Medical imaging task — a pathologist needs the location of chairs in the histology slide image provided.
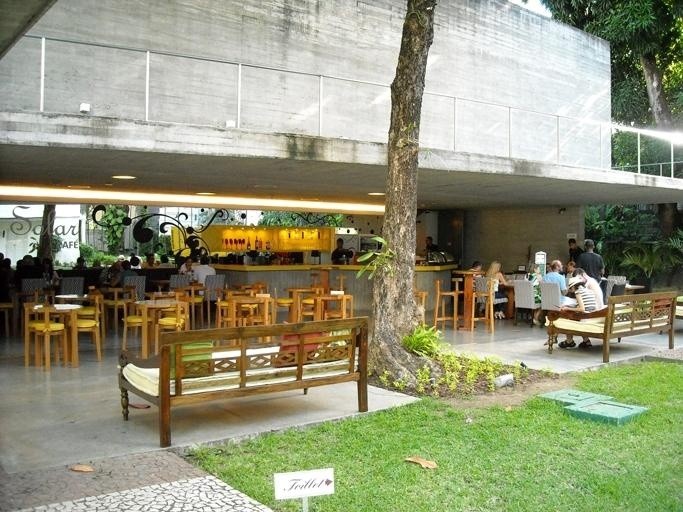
[0,274,355,373]
[471,275,627,334]
[434,280,459,332]
[414,282,429,327]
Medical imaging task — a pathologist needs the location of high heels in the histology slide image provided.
[494,311,505,319]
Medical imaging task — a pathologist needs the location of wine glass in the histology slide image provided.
[222,238,246,250]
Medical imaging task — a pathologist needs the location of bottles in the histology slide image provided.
[265,240,270,249]
[246,236,250,249]
[227,251,295,266]
[254,236,259,249]
[258,237,261,250]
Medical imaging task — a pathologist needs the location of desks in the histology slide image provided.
[454,270,486,330]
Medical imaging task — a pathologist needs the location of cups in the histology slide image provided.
[156,260,160,267]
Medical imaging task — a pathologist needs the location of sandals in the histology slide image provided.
[579,339,592,347]
[558,340,576,348]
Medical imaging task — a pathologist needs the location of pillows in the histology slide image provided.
[168,338,213,380]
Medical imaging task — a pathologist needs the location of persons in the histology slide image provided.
[426,236,439,261]
[0,248,216,320]
[524,238,606,350]
[485,259,512,320]
[331,238,353,264]
[467,260,485,317]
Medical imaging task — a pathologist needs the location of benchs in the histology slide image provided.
[118,317,369,447]
[547,291,679,363]
